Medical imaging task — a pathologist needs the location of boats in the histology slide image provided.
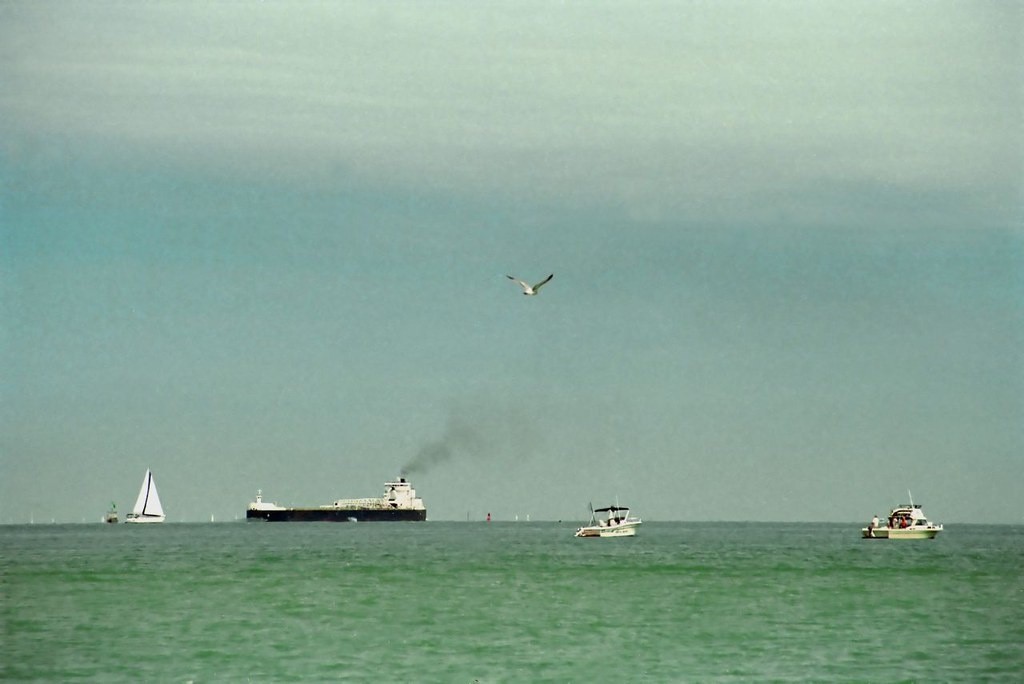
[105,502,119,524]
[573,494,643,537]
[245,476,427,522]
[861,489,944,539]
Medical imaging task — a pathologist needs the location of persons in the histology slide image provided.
[872,515,879,528]
[606,507,616,527]
[889,516,908,529]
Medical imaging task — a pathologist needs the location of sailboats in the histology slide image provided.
[125,466,166,524]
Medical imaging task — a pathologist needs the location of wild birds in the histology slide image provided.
[506,273,554,295]
